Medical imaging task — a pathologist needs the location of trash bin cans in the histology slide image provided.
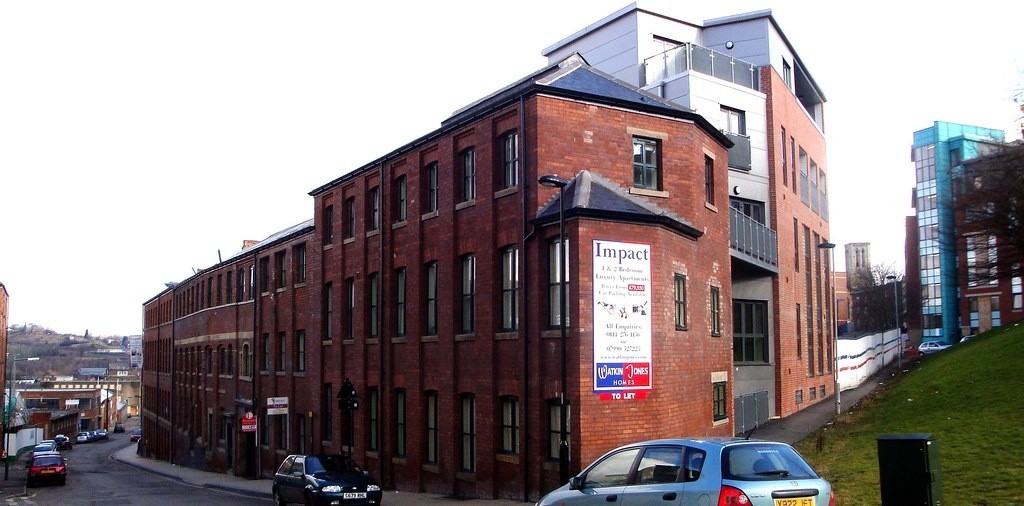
[875,432,941,506]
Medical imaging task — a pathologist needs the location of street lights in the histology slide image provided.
[886,275,902,369]
[4,357,40,481]
[817,243,841,415]
[537,175,570,487]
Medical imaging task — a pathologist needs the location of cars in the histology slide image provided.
[533,437,836,506]
[130,430,141,442]
[54,434,73,450]
[114,423,126,433]
[918,341,953,356]
[272,454,383,506]
[27,439,66,485]
[76,429,109,443]
